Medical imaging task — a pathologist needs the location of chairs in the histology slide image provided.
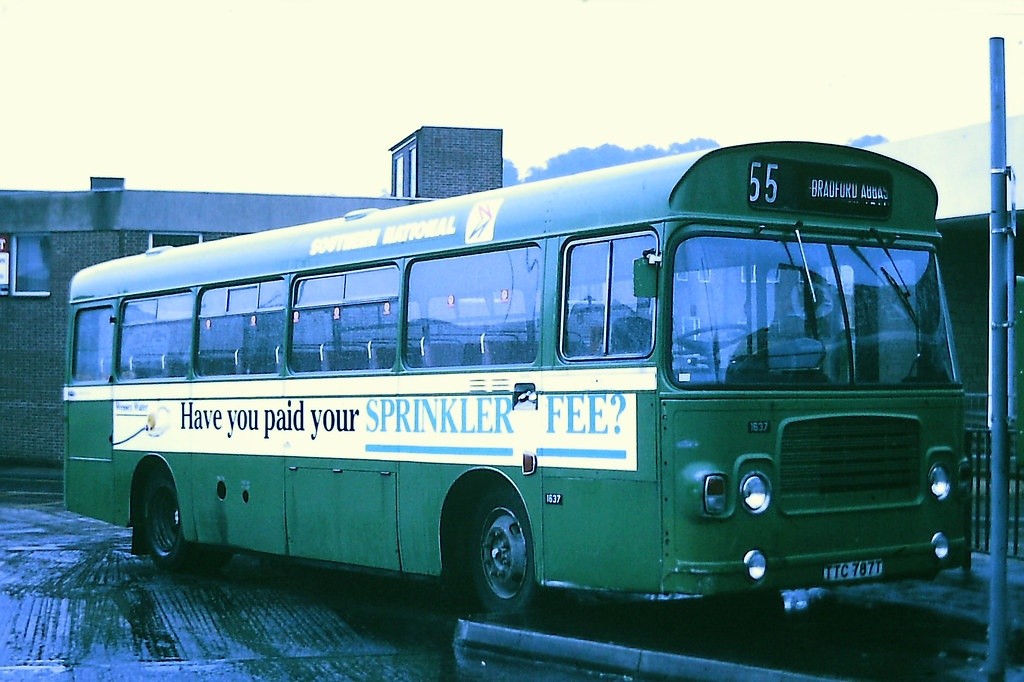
[99,292,667,381]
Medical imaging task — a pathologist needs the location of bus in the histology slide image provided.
[58,140,966,618]
